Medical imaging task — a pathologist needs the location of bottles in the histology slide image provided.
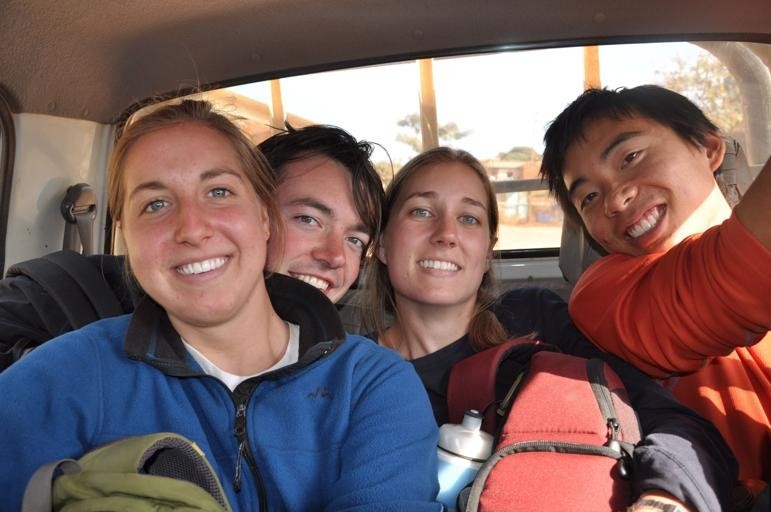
[429,410,495,511]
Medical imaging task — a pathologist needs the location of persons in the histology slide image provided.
[0,90,444,512]
[1,124,395,373]
[356,145,742,512]
[538,83,771,512]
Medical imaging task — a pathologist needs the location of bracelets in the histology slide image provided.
[637,497,682,512]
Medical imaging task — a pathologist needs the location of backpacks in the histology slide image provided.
[447,339,642,512]
[21,432,234,511]
